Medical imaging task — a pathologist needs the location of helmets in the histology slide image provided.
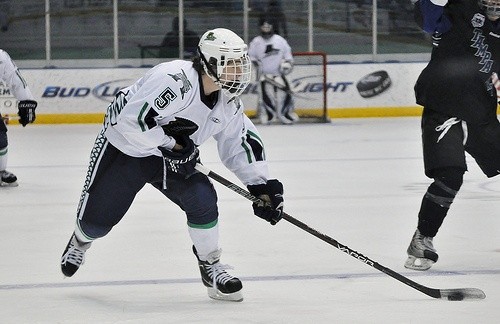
[198,28,251,97]
[258,18,275,40]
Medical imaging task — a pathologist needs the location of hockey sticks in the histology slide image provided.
[194,163,488,302]
[0,116,21,120]
[262,71,319,101]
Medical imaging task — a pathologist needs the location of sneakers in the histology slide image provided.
[0,170,18,188]
[404,230,439,269]
[192,245,244,303]
[61,232,92,276]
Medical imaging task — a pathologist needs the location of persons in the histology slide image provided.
[158,16,201,59]
[60,28,284,302]
[0,49,37,187]
[248,22,299,125]
[404,0,500,271]
[250,0,288,38]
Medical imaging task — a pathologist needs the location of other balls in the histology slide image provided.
[448,292,464,301]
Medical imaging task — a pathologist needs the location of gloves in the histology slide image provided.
[247,180,283,225]
[158,135,201,173]
[18,100,38,127]
